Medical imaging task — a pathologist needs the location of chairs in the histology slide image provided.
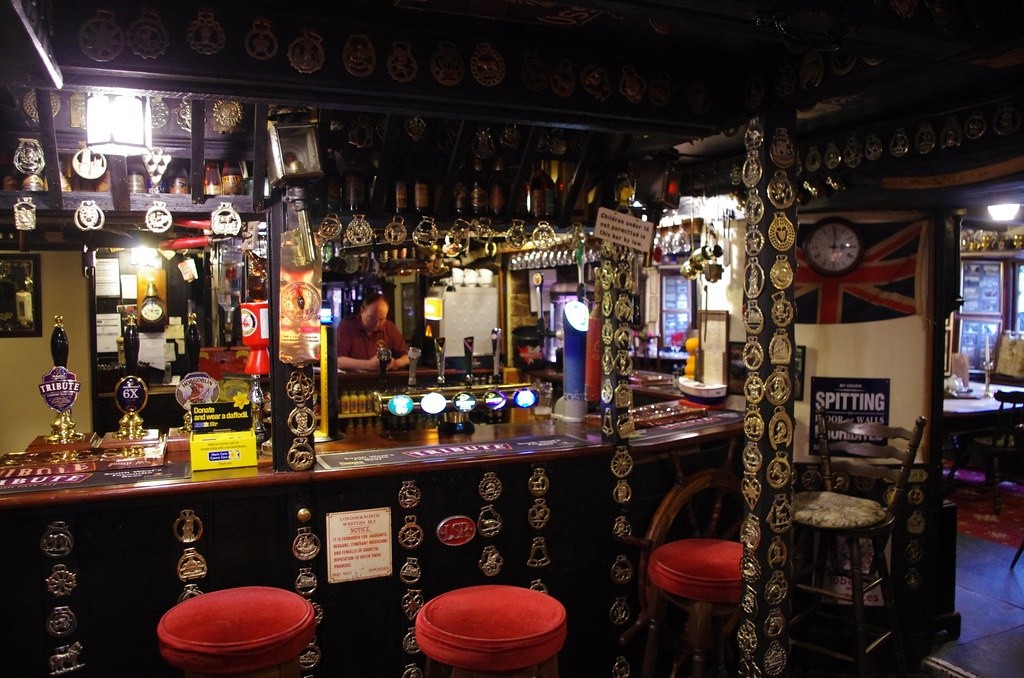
[632,334,663,372]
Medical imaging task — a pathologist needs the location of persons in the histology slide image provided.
[337,294,410,372]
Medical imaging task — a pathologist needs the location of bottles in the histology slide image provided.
[204,159,255,195]
[316,143,380,211]
[386,156,432,214]
[219,249,244,343]
[520,157,573,216]
[337,389,375,414]
[672,364,679,390]
[61,161,110,192]
[2,163,46,191]
[341,415,440,436]
[442,409,507,425]
[451,151,517,215]
[380,375,501,395]
[127,160,191,194]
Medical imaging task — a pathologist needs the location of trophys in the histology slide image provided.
[491,328,500,384]
[406,346,420,394]
[24,315,102,453]
[373,347,391,396]
[433,337,446,389]
[95,315,165,452]
[463,337,473,391]
[166,314,220,451]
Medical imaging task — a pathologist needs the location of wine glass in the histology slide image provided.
[652,218,691,256]
[508,244,578,271]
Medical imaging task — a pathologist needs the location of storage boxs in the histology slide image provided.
[189,428,258,472]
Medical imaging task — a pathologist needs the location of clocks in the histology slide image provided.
[801,216,866,278]
[140,298,166,324]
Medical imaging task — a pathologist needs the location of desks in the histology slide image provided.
[941,379,1024,452]
[631,351,687,375]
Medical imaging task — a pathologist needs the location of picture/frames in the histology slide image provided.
[957,260,1008,377]
[0,253,42,338]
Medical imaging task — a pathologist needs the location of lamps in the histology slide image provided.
[85,91,153,156]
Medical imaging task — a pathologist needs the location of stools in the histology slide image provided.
[789,413,928,678]
[416,585,567,678]
[943,391,1024,515]
[647,538,743,678]
[156,585,316,678]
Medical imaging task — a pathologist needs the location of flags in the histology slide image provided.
[793,219,924,324]
